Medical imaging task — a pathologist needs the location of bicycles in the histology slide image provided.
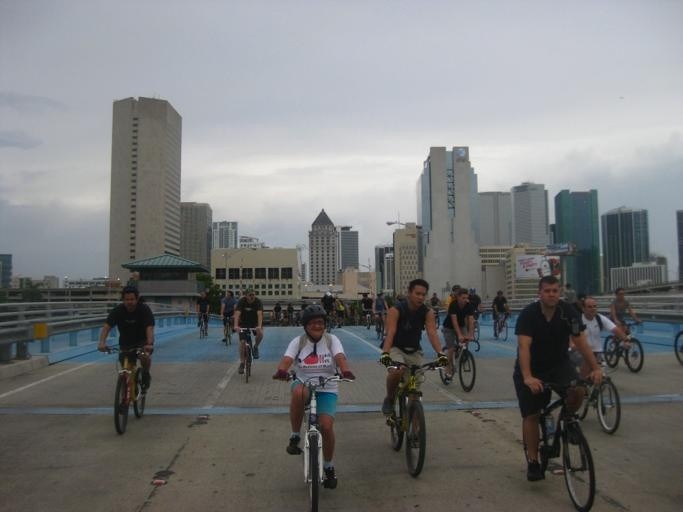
[605,320,644,373]
[378,357,440,476]
[194,310,209,340]
[494,313,510,341]
[432,309,488,394]
[269,309,388,341]
[97,344,152,434]
[231,328,256,383]
[270,373,354,512]
[220,315,234,346]
[522,345,626,511]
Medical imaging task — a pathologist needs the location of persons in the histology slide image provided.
[272,304,355,489]
[221,291,238,342]
[510,276,608,482]
[273,285,512,375]
[195,290,210,336]
[565,283,646,377]
[377,278,450,417]
[97,285,155,414]
[233,288,264,374]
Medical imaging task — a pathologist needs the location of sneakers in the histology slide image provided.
[382,398,393,413]
[253,345,259,359]
[324,466,337,489]
[287,435,302,455]
[564,423,583,444]
[239,363,245,373]
[527,459,545,481]
[141,372,150,388]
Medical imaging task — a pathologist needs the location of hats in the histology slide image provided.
[246,288,255,296]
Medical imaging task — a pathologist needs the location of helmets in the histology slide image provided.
[301,304,327,324]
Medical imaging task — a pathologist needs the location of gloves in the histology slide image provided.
[380,352,391,367]
[438,352,450,366]
[342,371,355,380]
[277,369,288,378]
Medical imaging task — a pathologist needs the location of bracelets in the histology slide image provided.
[144,342,153,345]
